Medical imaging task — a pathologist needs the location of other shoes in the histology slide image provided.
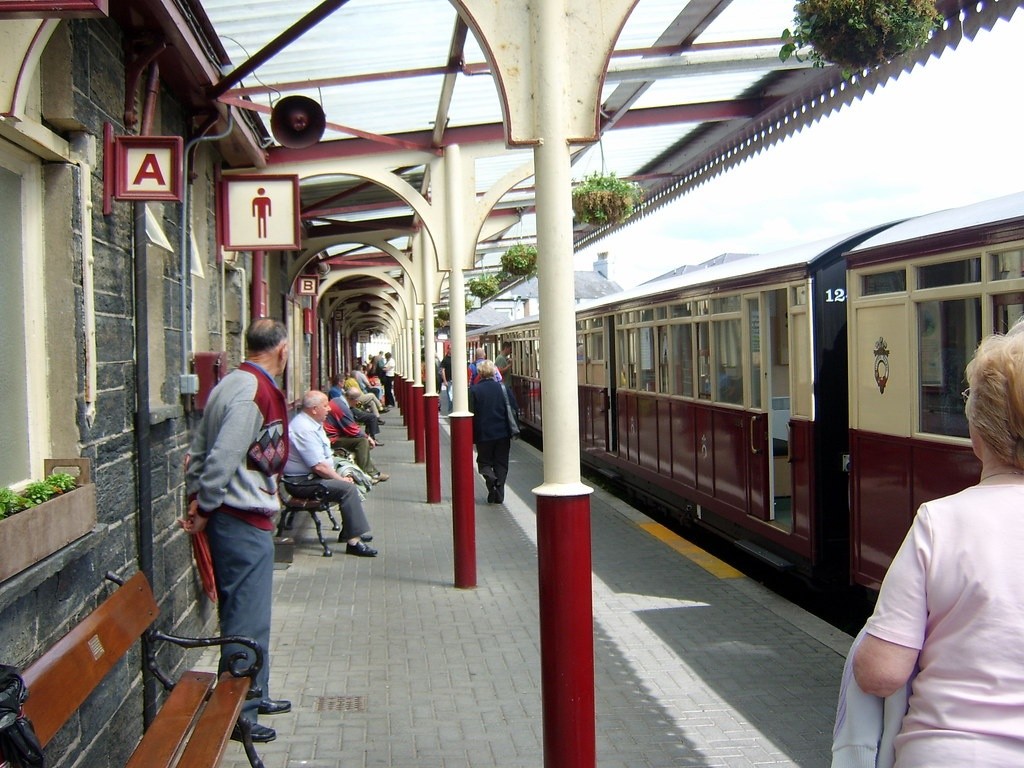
[488,485,497,503]
[375,441,384,446]
[374,473,390,481]
[370,478,379,485]
[378,419,384,425]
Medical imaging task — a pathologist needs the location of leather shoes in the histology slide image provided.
[258,698,291,714]
[360,534,373,542]
[230,723,276,742]
[346,541,378,556]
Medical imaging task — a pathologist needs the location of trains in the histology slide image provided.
[443,189,1024,596]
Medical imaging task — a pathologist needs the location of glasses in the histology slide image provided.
[962,387,972,403]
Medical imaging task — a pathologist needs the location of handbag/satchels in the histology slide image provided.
[502,383,520,436]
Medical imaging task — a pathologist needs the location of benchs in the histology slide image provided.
[0,571,263,768]
[276,448,347,556]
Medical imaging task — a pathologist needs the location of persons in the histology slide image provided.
[283,390,378,557]
[382,352,395,407]
[356,351,386,373]
[421,346,453,414]
[468,359,519,504]
[467,347,486,388]
[352,365,382,402]
[178,319,291,742]
[852,308,1024,768]
[362,366,388,410]
[344,370,391,424]
[322,387,390,486]
[495,342,526,430]
[327,374,384,446]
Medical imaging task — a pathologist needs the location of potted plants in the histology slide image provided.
[778,0,945,88]
[500,244,538,280]
[572,171,644,225]
[469,276,500,298]
[0,458,96,582]
